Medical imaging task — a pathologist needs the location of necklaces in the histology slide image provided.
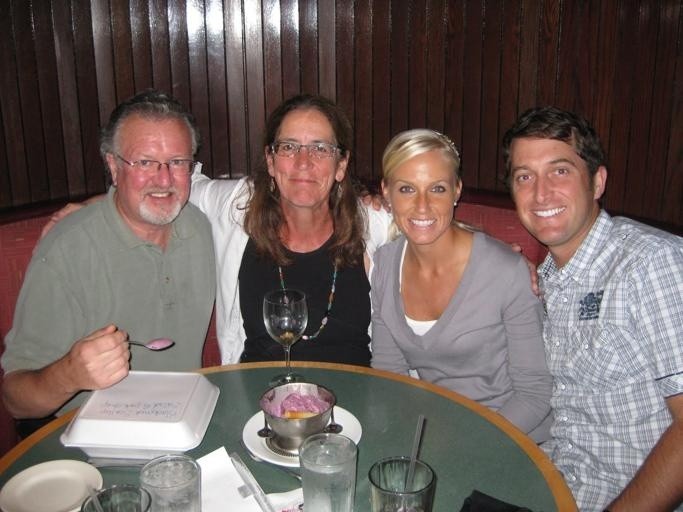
[275,262,339,341]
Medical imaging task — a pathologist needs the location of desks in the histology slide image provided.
[0,354,579,510]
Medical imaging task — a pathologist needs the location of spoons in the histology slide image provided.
[127,338,176,351]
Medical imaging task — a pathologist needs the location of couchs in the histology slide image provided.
[374,194,550,275]
[0,192,222,469]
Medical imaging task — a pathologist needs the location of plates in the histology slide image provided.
[242,406,361,468]
[0,459,102,512]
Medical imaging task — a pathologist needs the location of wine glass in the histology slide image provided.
[263,289,309,388]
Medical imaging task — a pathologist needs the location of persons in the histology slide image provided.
[0,90,391,423]
[39,91,541,433]
[368,129,552,463]
[503,105,683,512]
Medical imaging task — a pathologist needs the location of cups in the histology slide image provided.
[259,382,337,451]
[297,431,359,511]
[80,482,151,512]
[136,453,202,511]
[367,455,437,512]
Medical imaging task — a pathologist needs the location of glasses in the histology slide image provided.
[113,152,199,175]
[270,141,343,160]
